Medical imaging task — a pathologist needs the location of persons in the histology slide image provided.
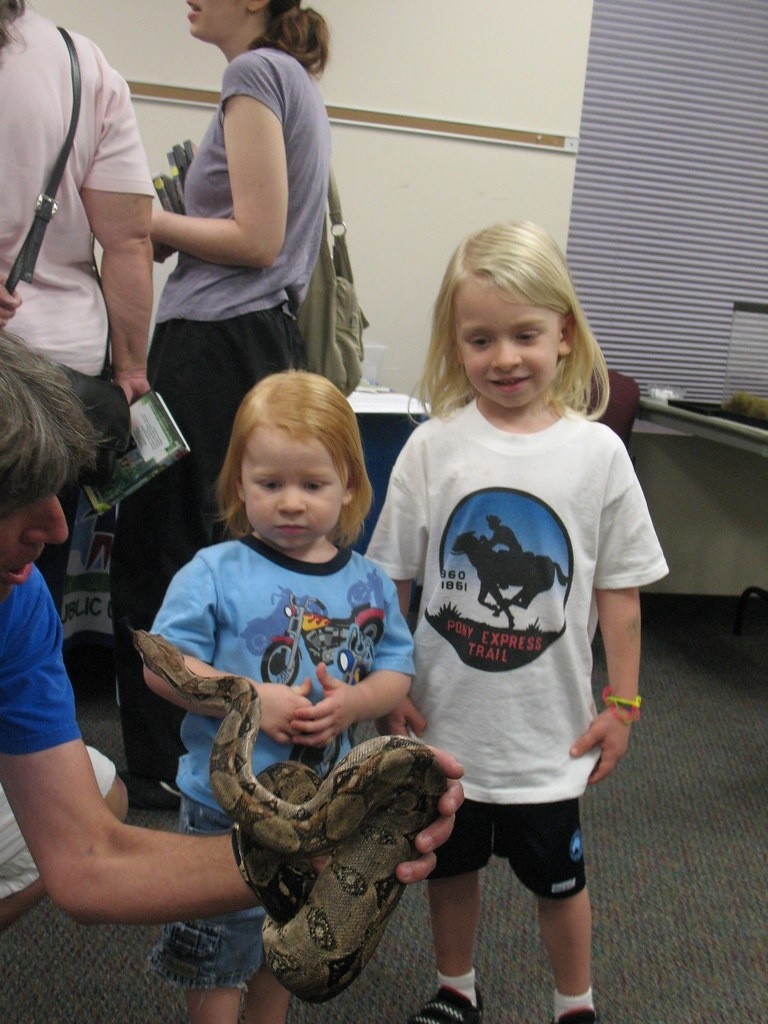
[143,363,415,1023]
[113,0,328,812]
[365,215,670,1024]
[0,0,156,602]
[0,321,466,1024]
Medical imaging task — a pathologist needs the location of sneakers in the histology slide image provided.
[408,983,482,1024]
[553,1007,595,1024]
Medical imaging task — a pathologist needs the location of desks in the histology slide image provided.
[634,397,768,636]
[40,392,433,701]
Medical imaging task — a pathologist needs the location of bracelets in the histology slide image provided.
[602,687,644,723]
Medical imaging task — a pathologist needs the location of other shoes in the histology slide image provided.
[117,771,180,810]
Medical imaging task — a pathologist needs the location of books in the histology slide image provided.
[81,391,190,517]
[154,137,197,219]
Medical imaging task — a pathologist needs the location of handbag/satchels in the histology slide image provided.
[49,362,129,489]
[296,276,370,398]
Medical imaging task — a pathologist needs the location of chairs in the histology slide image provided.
[587,368,640,447]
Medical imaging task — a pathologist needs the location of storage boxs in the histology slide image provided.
[362,332,392,385]
